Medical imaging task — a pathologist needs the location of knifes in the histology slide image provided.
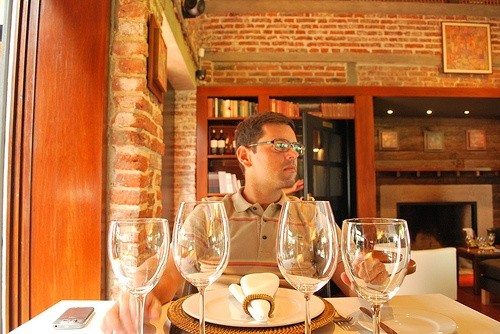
[359,307,398,334]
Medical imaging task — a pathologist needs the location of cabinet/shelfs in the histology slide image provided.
[205,96,355,196]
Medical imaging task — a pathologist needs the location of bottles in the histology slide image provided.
[217,129,225,154]
[209,129,217,154]
[232,130,237,154]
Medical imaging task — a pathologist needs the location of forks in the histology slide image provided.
[332,310,354,323]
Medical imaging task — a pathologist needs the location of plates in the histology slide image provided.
[182,285,325,329]
[357,306,458,334]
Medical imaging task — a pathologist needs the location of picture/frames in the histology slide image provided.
[153,28,168,93]
[441,22,492,74]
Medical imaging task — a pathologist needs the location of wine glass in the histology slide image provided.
[342,216,410,334]
[109,218,170,334]
[276,200,339,333]
[171,200,230,334]
[465,233,495,252]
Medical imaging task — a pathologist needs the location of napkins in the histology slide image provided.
[229,273,279,322]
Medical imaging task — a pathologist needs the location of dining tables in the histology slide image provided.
[9,247,500,334]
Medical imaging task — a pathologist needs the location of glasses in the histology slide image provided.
[245,138,305,156]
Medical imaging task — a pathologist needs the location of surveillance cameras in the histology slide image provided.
[185,0,206,16]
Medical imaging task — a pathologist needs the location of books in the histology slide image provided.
[307,102,355,118]
[207,98,300,118]
[208,170,242,194]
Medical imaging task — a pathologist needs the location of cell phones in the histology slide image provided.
[54,307,94,329]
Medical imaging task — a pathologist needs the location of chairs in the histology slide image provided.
[374,247,459,300]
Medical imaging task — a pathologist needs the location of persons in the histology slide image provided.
[281,178,304,194]
[100,112,388,334]
